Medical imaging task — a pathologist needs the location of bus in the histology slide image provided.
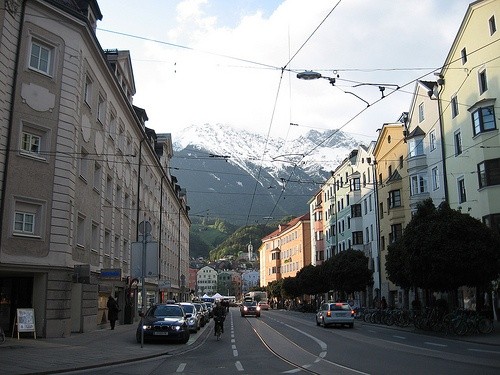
[244,292,267,303]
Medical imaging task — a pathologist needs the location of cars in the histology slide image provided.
[175,303,201,333]
[240,302,260,317]
[200,303,210,325]
[316,303,355,329]
[205,302,214,319]
[258,302,269,310]
[192,303,207,329]
[136,304,189,344]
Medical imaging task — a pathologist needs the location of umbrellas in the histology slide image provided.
[198,293,228,302]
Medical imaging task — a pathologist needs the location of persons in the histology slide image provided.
[106,295,120,330]
[212,303,226,336]
[434,294,448,313]
[173,295,177,303]
[220,299,230,313]
[412,295,422,311]
[463,296,471,312]
[270,299,274,305]
[380,296,388,310]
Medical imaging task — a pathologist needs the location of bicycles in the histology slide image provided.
[214,315,225,342]
[364,307,413,327]
[415,306,492,337]
